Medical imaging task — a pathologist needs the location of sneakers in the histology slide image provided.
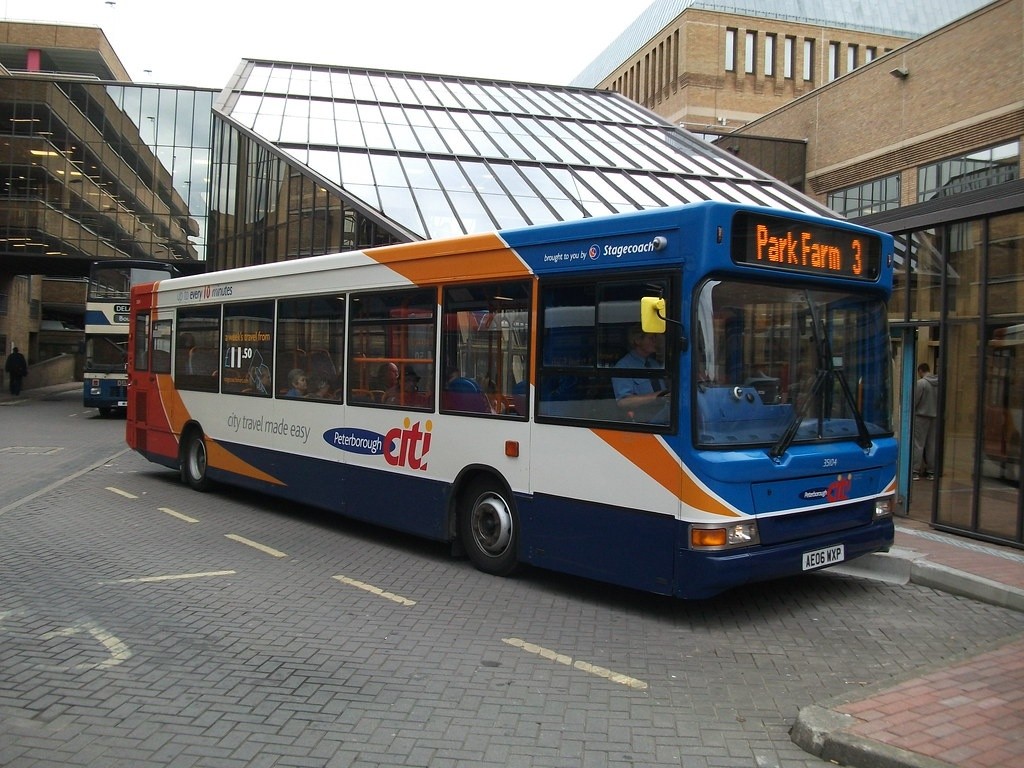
[912,473,920,481]
[925,473,935,480]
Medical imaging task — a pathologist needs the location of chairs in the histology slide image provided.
[449,377,485,413]
[306,348,337,383]
[190,345,217,375]
[277,348,306,369]
[512,380,526,416]
[141,349,169,369]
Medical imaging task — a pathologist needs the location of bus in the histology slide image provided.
[83,260,185,416]
[975,324,1024,464]
[126,202,899,600]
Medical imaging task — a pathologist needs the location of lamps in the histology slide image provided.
[889,68,909,78]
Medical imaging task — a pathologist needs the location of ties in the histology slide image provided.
[644,361,662,392]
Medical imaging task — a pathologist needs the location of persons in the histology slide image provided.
[175,334,194,374]
[349,351,369,377]
[248,366,271,394]
[373,363,399,392]
[914,363,938,481]
[286,369,308,398]
[611,323,672,422]
[396,366,422,407]
[6,347,27,395]
[308,373,330,400]
